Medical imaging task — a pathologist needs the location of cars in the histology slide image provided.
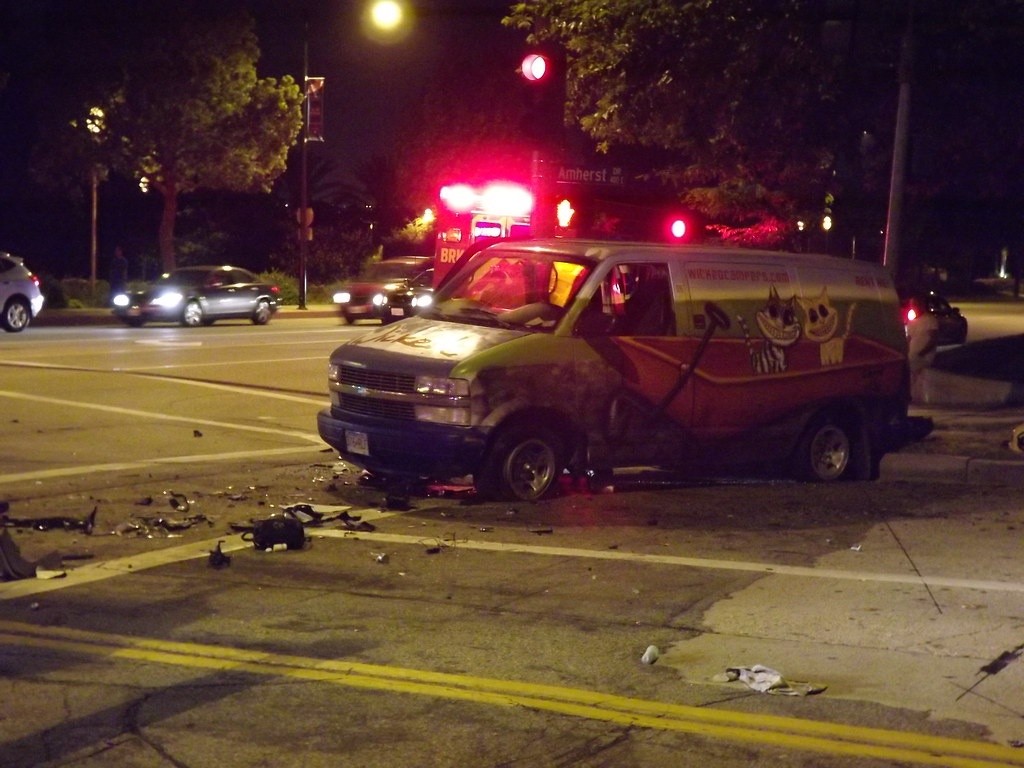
[112,263,280,328]
[328,256,437,326]
[893,291,968,357]
[1,252,45,331]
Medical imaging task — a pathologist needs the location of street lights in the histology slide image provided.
[86,104,106,295]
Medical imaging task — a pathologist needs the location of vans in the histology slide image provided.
[313,240,911,507]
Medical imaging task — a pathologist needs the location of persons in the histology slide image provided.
[106,245,129,309]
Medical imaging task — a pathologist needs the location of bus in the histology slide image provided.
[424,131,703,298]
